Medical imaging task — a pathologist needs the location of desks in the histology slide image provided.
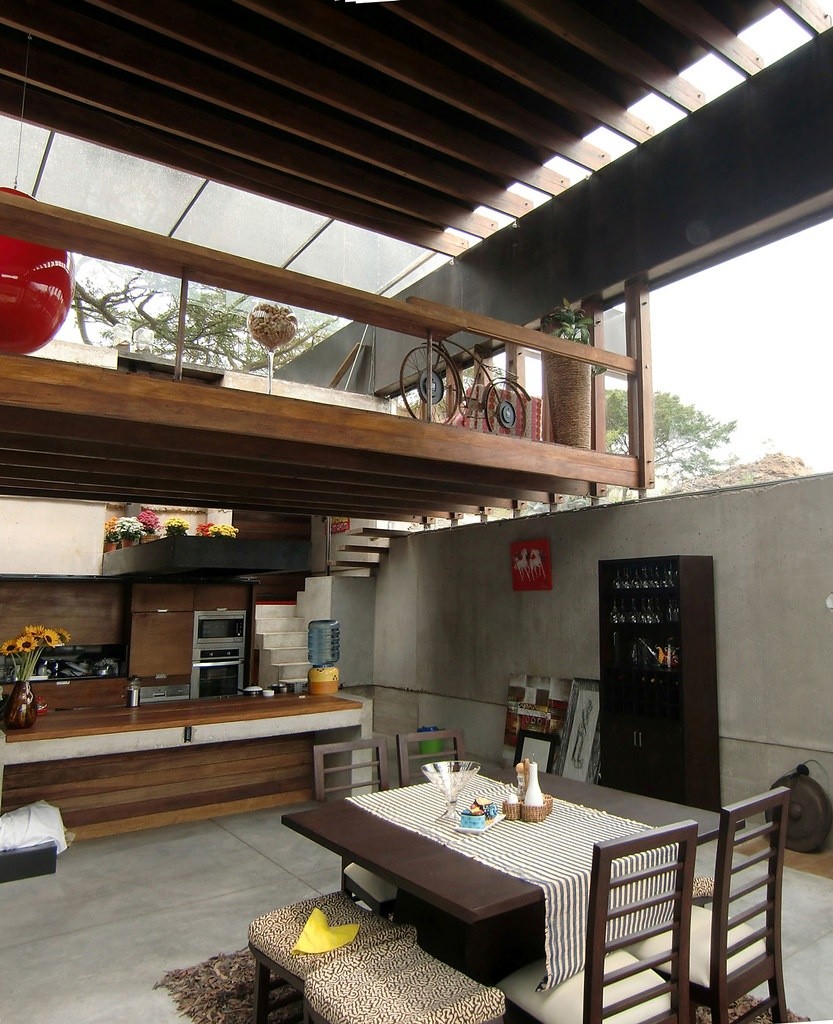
[284,768,680,989]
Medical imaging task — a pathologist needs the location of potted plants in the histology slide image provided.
[540,298,609,450]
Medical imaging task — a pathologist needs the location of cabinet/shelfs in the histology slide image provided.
[596,554,720,813]
[0,677,130,714]
[129,580,247,676]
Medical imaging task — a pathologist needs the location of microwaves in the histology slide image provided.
[192,610,246,659]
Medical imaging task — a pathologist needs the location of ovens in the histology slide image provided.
[191,660,244,699]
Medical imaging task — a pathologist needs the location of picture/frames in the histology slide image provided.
[512,676,604,786]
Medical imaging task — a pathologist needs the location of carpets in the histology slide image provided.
[154,945,814,1024]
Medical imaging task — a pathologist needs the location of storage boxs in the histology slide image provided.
[0,841,58,883]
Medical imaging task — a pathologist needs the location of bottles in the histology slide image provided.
[307,665,339,695]
[516,763,525,802]
[129,677,141,689]
[525,762,543,806]
[307,620,340,664]
[36,696,48,715]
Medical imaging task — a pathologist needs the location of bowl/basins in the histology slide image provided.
[262,690,274,696]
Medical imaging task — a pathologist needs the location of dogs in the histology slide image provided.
[515,549,545,582]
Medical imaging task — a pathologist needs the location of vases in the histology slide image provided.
[139,533,158,544]
[104,543,117,553]
[3,680,39,730]
[121,540,133,549]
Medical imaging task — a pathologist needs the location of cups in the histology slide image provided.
[127,689,140,708]
[37,666,47,676]
[418,739,442,754]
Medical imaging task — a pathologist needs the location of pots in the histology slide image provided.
[235,687,262,695]
[271,684,294,694]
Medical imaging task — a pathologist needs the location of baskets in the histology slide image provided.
[502,794,554,822]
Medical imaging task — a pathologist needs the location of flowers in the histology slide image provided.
[106,507,239,550]
[0,624,71,681]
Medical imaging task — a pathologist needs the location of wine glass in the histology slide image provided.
[421,761,481,821]
[610,564,680,625]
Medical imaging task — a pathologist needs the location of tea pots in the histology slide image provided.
[635,636,681,669]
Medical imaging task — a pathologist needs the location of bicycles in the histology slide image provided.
[398,336,530,440]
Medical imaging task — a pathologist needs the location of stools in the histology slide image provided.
[248,891,505,1024]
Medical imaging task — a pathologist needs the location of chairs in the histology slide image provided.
[312,729,466,916]
[438,384,542,442]
[500,787,790,1024]
